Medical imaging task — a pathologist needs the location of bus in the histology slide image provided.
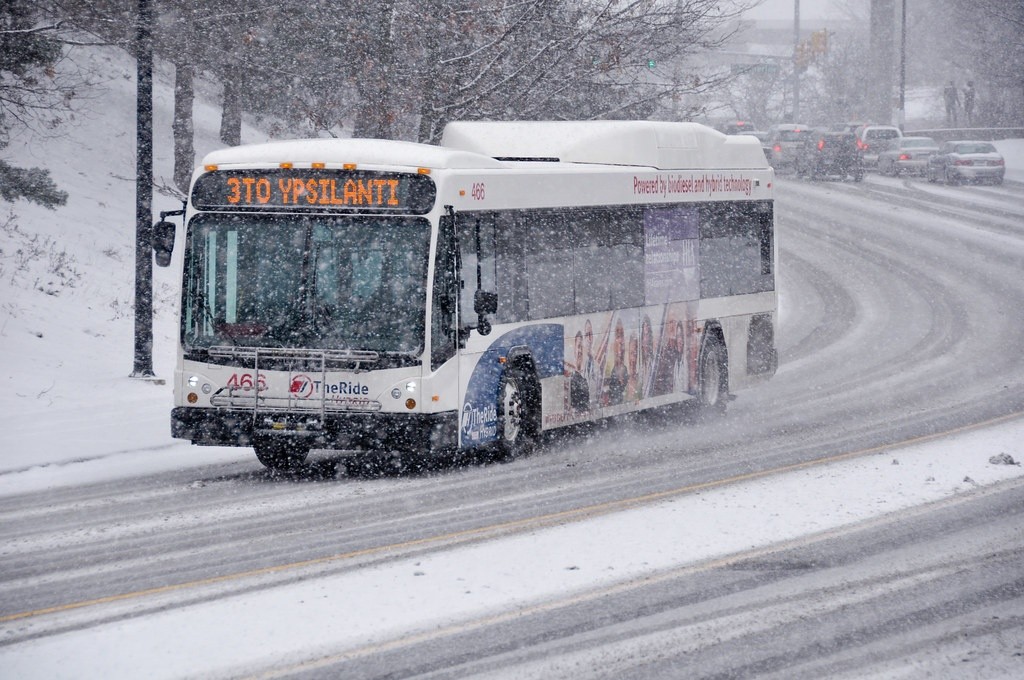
[150,119,780,473]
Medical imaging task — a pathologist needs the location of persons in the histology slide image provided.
[567,313,689,412]
[944,80,975,128]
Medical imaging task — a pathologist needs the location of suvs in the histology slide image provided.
[723,122,1006,186]
